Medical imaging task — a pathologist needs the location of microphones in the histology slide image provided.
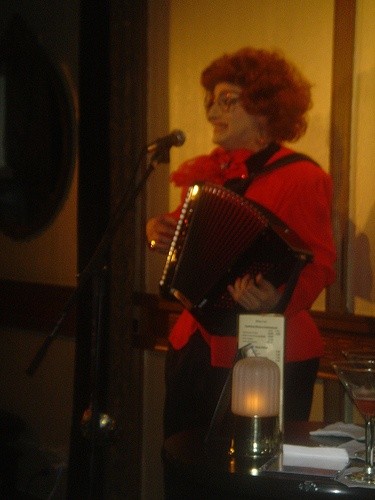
[143,129,186,153]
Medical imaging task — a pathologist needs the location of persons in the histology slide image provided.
[145,48,338,499]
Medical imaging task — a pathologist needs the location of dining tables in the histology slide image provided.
[162,421,375,499]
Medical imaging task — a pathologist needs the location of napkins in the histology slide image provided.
[282,444,349,471]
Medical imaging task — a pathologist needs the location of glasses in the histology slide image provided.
[204,90,241,112]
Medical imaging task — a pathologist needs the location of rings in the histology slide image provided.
[150,240,156,250]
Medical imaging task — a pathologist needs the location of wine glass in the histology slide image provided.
[330,357,375,486]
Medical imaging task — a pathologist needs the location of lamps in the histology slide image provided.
[231,342,280,457]
